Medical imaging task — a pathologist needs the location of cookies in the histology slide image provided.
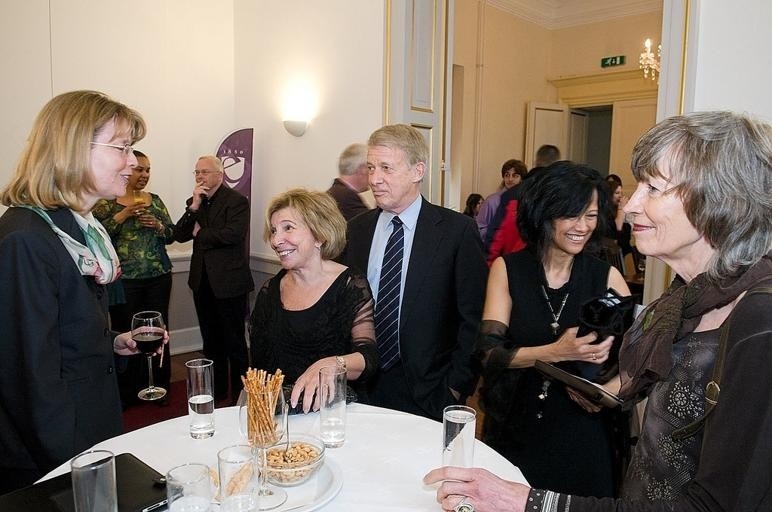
[239,365,285,447]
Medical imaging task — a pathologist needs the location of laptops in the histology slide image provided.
[0,452,184,512]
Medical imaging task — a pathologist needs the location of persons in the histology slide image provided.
[174,156,258,408]
[91,150,175,410]
[0,90,170,494]
[474,159,650,500]
[246,189,382,417]
[333,124,485,420]
[325,143,370,219]
[463,145,632,271]
[423,110,770,511]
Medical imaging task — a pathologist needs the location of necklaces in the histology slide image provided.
[539,283,569,331]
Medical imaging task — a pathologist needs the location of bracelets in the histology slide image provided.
[159,221,164,234]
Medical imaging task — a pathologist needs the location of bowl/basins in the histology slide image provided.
[258,433,326,489]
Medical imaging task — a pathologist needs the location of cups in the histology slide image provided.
[319,366,348,448]
[441,405,478,468]
[165,463,214,511]
[217,443,259,511]
[185,358,216,439]
[70,450,118,511]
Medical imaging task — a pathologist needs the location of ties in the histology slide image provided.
[376,217,404,372]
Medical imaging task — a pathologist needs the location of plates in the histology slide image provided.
[228,459,344,511]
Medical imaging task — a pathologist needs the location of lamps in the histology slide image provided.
[639,38,662,85]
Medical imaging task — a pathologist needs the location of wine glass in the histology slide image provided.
[131,309,167,402]
[134,190,145,227]
[237,386,288,511]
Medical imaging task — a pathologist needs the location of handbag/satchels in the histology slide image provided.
[577,289,637,384]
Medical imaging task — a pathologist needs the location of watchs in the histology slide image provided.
[334,354,348,371]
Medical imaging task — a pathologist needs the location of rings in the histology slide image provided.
[452,496,475,511]
[150,221,152,223]
[591,351,597,359]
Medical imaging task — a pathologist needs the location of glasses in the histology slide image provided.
[192,169,219,177]
[90,142,134,158]
